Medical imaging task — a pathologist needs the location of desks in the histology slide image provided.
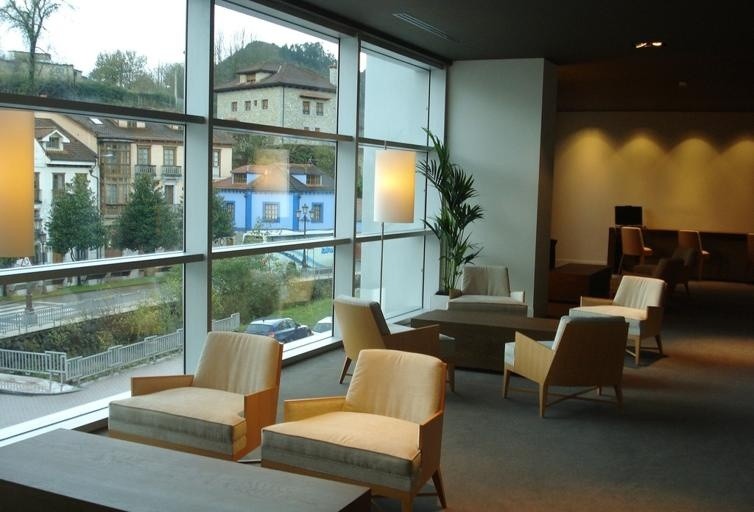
[1,426,370,511]
[410,308,559,373]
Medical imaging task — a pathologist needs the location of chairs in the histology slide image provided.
[333,295,456,384]
[617,225,721,278]
[107,331,284,463]
[447,265,531,316]
[502,275,668,418]
[260,349,447,511]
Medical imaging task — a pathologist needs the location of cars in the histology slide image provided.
[250,316,332,344]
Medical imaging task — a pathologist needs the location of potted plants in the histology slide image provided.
[416,126,486,298]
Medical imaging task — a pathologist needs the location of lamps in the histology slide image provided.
[0,108,36,260]
[373,138,415,309]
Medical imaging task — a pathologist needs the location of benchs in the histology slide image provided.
[547,262,611,319]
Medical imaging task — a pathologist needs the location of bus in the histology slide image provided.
[241,228,335,275]
